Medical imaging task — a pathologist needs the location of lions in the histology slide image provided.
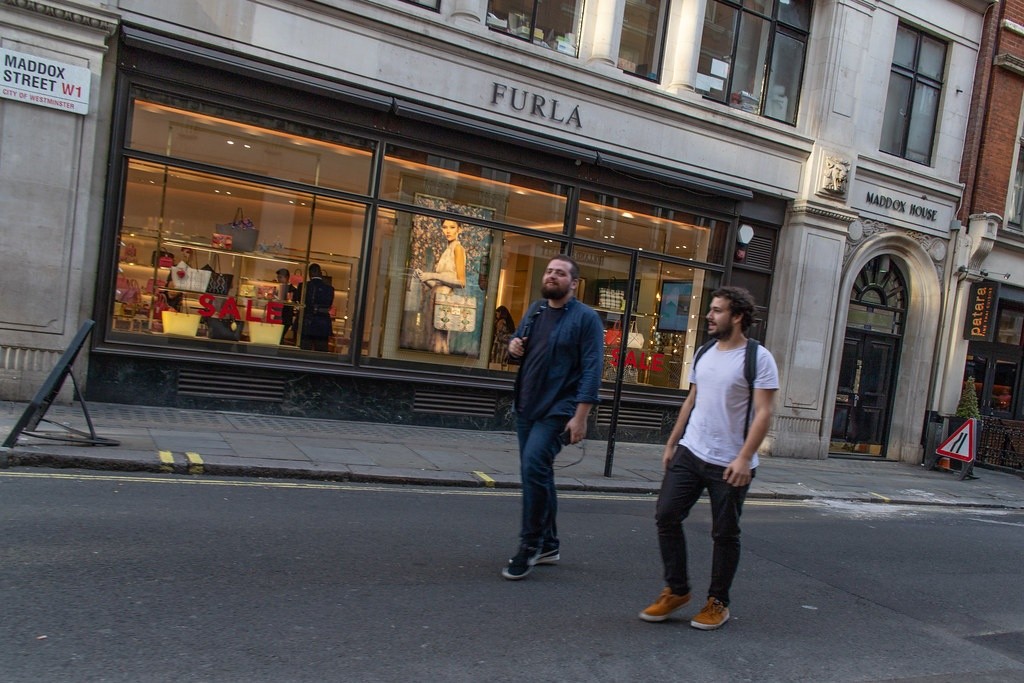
[823,155,852,196]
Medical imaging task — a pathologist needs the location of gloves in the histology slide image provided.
[420,272,460,288]
[413,269,435,289]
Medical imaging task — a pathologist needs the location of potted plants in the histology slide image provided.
[947,376,983,473]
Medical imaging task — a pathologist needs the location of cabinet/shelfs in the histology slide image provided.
[112,226,359,355]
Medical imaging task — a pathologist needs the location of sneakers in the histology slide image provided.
[508,547,560,564]
[505,541,542,578]
[690,597,730,630]
[638,586,693,621]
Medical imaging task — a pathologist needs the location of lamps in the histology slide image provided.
[980,269,1011,280]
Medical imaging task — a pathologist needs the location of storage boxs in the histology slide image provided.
[619,58,637,72]
[513,26,543,45]
[547,33,576,56]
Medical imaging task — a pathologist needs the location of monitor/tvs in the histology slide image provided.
[657,282,692,333]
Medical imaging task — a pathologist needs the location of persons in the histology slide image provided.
[639,287,779,630]
[180,247,193,267]
[502,254,603,578]
[277,264,334,352]
[159,271,183,312]
[412,219,467,355]
[489,305,516,365]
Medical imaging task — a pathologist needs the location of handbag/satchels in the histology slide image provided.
[171,249,211,293]
[321,269,333,285]
[599,277,624,311]
[261,287,273,299]
[203,300,245,342]
[606,321,621,346]
[432,283,477,333]
[216,206,258,253]
[201,253,234,296]
[238,284,256,297]
[115,240,183,319]
[289,269,303,285]
[626,321,644,349]
[161,296,201,337]
[605,354,638,384]
[248,321,285,345]
[212,233,232,251]
